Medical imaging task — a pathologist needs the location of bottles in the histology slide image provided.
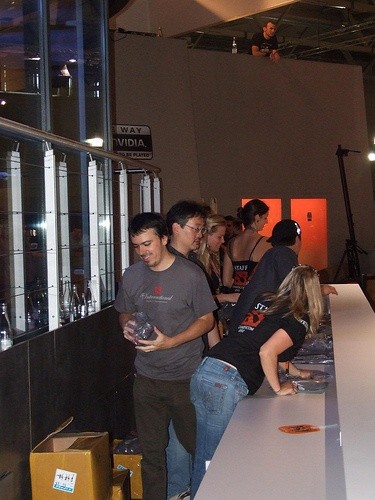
[0,303,13,353]
[232,36,237,53]
[58,280,96,323]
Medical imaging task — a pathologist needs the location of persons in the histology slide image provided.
[190,264,322,500]
[160,199,336,346]
[114,211,214,500]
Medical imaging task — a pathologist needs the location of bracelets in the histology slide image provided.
[285,360,300,379]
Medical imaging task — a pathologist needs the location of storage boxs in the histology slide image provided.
[30,416,143,500]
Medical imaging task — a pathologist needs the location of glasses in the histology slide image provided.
[184,223,207,234]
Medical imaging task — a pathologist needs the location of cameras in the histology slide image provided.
[128,313,153,345]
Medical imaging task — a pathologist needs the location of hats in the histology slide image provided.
[266,219,301,243]
